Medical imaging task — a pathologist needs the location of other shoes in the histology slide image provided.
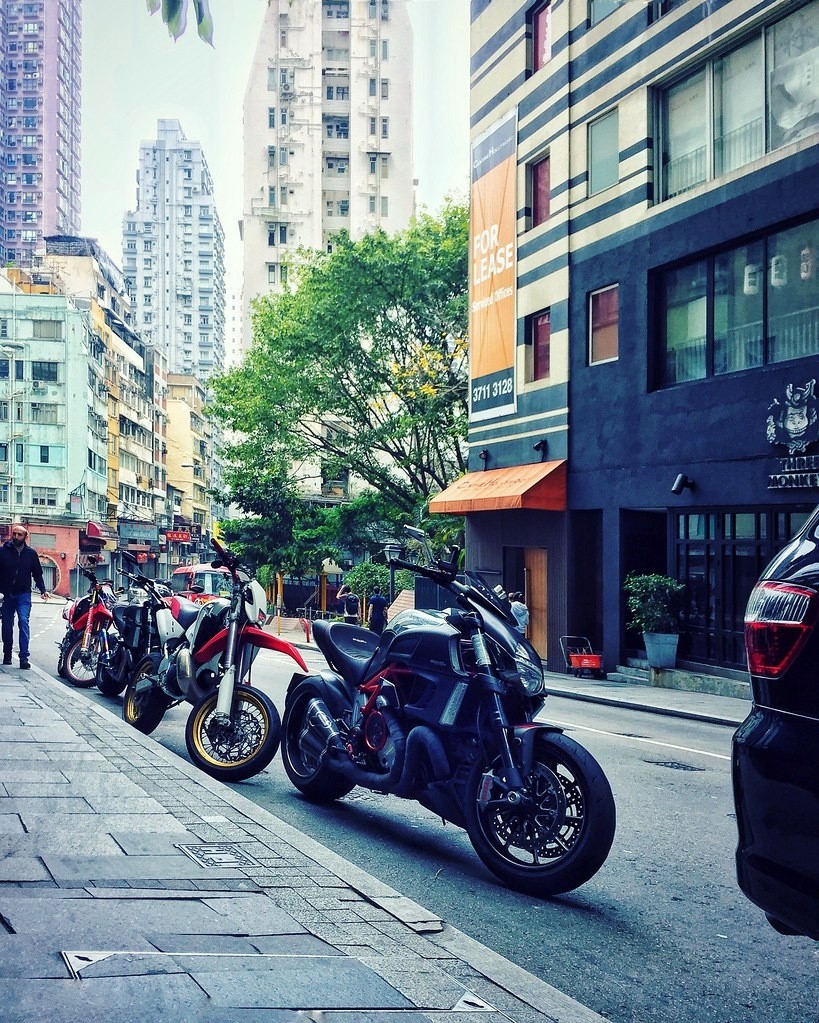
[3,654,12,665]
[20,660,30,669]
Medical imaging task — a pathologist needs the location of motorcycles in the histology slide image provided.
[280,524,616,900]
[54,536,309,784]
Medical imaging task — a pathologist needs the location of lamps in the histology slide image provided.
[61,552,66,559]
[533,439,545,451]
[478,450,487,459]
[670,474,693,494]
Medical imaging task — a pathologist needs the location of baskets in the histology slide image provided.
[569,654,602,668]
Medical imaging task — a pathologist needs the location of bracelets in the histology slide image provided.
[340,589,342,591]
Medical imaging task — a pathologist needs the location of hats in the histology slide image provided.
[494,584,503,593]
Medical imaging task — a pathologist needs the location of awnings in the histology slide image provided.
[428,459,568,515]
[88,522,118,538]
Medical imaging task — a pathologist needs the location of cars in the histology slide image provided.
[128,579,150,601]
[730,507,818,939]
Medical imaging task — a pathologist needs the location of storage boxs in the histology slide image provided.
[568,653,602,668]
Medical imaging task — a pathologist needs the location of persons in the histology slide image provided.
[508,593,528,637]
[336,585,361,624]
[0,526,51,668]
[367,586,388,635]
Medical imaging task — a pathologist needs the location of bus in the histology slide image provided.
[171,564,265,626]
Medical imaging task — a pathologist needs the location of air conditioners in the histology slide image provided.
[37,193,42,198]
[37,117,42,122]
[38,156,42,159]
[268,223,275,231]
[37,174,42,179]
[37,230,43,235]
[38,80,42,85]
[37,4,43,65]
[38,98,43,103]
[17,4,22,253]
[37,136,43,140]
[37,212,41,216]
[32,380,45,389]
[282,83,293,93]
[192,186,199,191]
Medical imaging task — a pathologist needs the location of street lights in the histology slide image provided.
[183,464,212,545]
[383,544,402,605]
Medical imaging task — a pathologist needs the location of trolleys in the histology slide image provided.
[559,636,603,678]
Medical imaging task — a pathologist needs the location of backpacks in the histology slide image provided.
[345,594,359,615]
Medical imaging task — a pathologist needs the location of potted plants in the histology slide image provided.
[622,571,687,669]
